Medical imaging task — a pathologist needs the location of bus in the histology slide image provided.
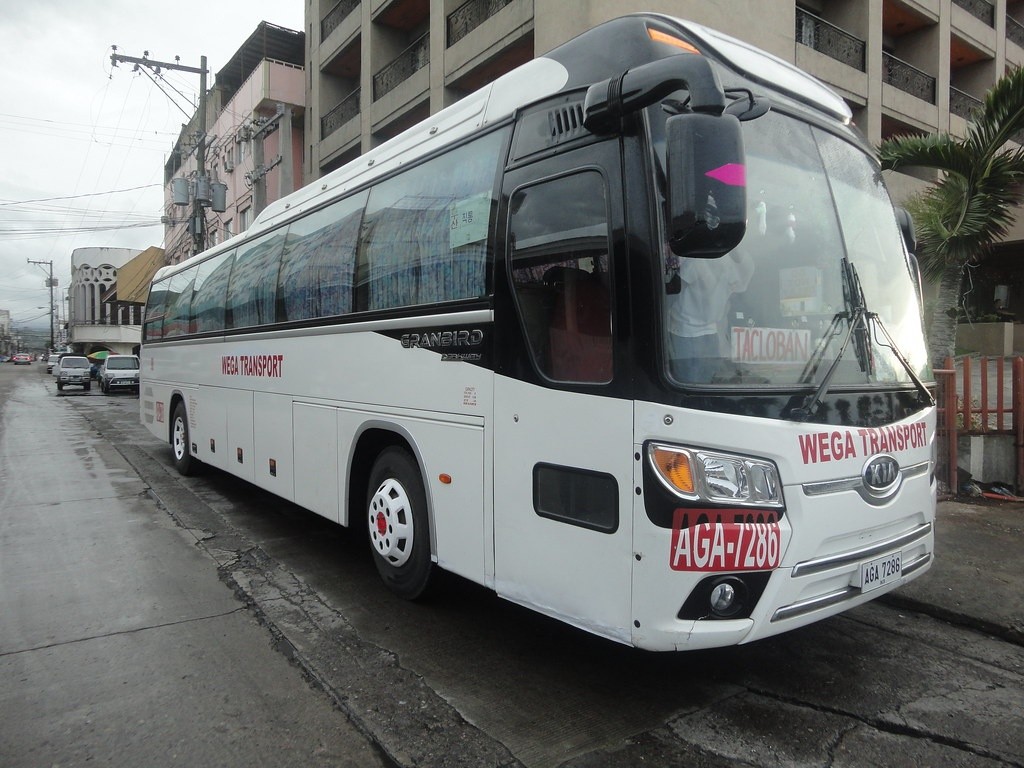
[139,12,938,651]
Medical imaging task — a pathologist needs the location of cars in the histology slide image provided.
[48,354,60,374]
[14,353,31,365]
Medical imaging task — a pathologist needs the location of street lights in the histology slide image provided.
[37,306,54,353]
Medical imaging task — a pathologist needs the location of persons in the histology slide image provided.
[663,242,720,383]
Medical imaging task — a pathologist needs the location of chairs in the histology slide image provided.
[540,266,612,379]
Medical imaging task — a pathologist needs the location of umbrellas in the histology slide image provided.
[86,351,119,363]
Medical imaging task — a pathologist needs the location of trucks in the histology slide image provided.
[100,355,140,396]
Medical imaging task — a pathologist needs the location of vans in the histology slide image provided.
[56,355,94,392]
